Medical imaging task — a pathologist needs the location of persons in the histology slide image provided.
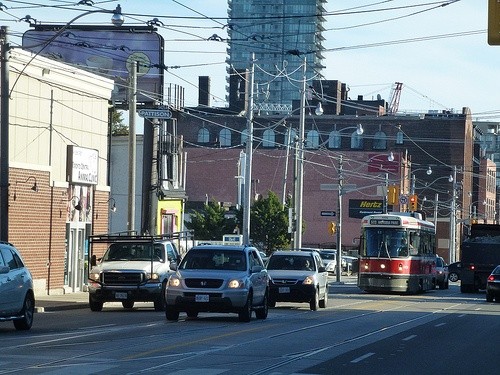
[304,259,313,271]
[397,238,413,251]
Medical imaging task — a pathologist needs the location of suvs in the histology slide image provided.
[87,239,183,313]
[164,244,270,322]
[265,250,329,311]
[0,241,38,332]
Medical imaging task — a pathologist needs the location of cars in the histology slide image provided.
[485,265,500,303]
[449,262,461,282]
[302,248,347,274]
[436,257,449,289]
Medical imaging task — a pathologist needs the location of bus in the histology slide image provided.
[353,212,436,296]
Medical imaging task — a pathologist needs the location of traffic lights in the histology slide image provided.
[408,195,417,211]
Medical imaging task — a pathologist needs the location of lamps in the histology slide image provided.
[13,176,38,201]
[96,198,117,219]
[60,195,83,218]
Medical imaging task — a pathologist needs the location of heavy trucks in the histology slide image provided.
[461,223,500,293]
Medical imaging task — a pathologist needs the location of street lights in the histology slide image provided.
[293,123,364,253]
[0,3,125,245]
[236,102,324,207]
[383,167,497,228]
[336,151,394,283]
